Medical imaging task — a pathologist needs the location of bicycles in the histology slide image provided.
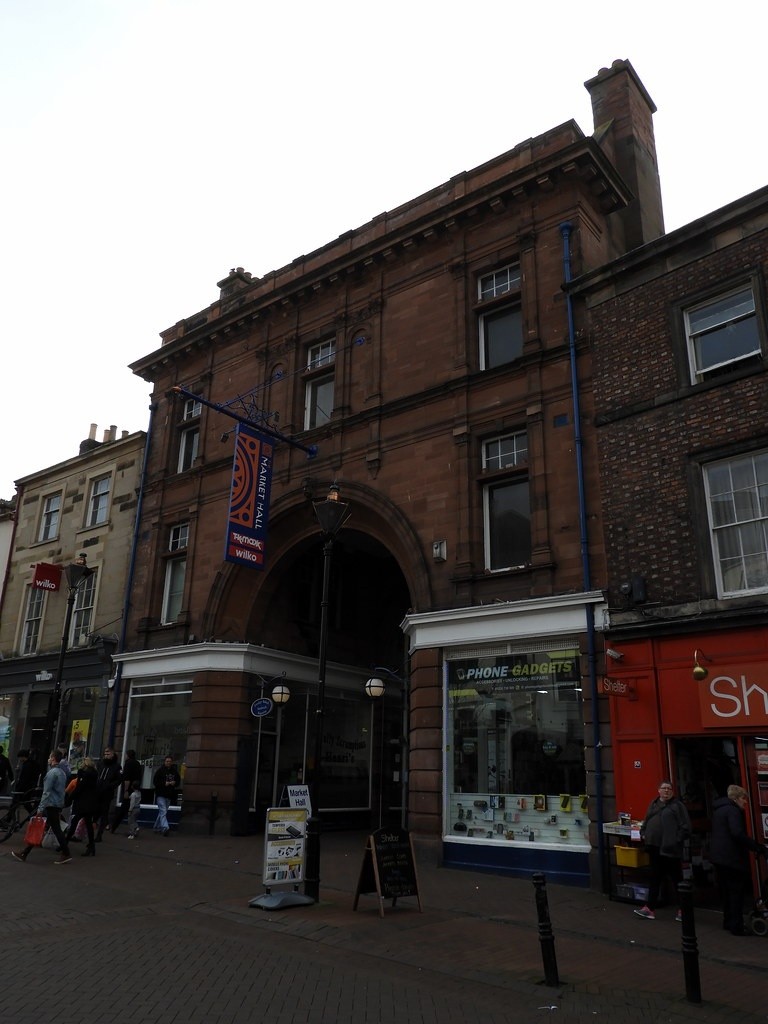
[0,784,42,844]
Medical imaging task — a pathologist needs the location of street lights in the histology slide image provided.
[304,478,353,901]
[35,552,96,793]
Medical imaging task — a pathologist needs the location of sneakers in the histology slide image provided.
[54,855,72,864]
[633,906,654,919]
[12,851,25,862]
[128,827,140,841]
[676,910,681,921]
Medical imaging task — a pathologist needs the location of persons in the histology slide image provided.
[1,745,16,795]
[110,750,141,834]
[705,785,767,937]
[0,750,37,829]
[12,749,73,864]
[153,756,180,837]
[70,749,123,843]
[125,781,142,839]
[47,743,70,784]
[56,757,102,857]
[632,780,693,922]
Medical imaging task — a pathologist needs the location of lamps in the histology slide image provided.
[271,683,291,707]
[365,677,387,699]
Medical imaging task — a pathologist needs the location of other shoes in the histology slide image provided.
[153,830,173,837]
[733,928,753,936]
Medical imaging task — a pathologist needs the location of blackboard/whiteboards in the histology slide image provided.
[356,825,418,899]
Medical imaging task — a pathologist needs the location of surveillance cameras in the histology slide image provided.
[607,646,624,661]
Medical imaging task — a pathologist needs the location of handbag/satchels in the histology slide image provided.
[24,813,47,846]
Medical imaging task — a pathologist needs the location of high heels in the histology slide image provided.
[82,842,95,857]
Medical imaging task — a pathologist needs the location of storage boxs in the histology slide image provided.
[616,882,650,901]
[613,844,649,869]
